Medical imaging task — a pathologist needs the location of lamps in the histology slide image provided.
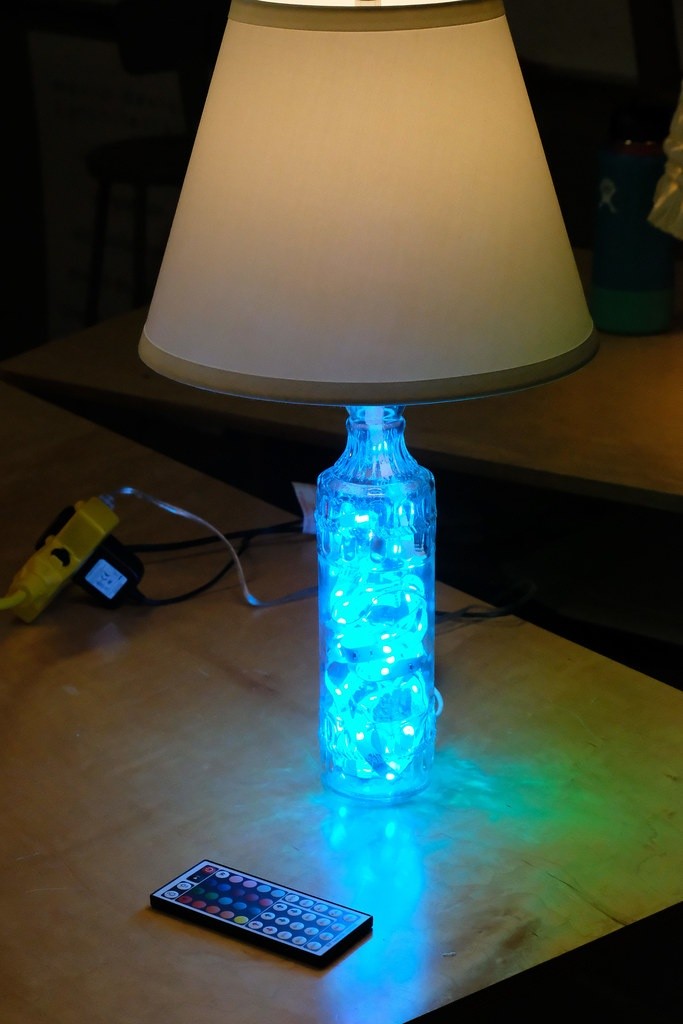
[137,0,598,808]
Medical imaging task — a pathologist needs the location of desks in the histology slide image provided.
[0,375,683,1024]
[0,248,683,516]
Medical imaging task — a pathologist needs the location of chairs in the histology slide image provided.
[81,0,217,325]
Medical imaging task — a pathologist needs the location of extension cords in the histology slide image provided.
[9,497,119,624]
